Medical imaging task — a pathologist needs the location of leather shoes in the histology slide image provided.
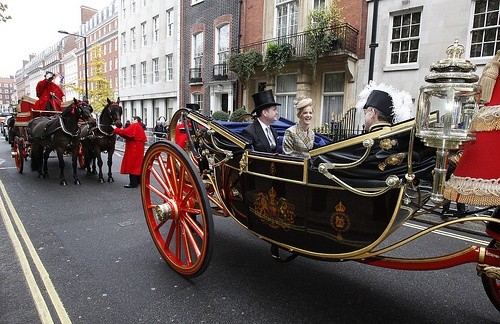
[124,183,137,188]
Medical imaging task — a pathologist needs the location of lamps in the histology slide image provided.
[415,38,482,204]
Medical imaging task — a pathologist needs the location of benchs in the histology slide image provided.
[220,123,327,152]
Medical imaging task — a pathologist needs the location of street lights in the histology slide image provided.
[58,29,88,102]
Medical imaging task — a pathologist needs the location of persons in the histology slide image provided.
[241,89,282,153]
[442,150,468,223]
[282,97,316,160]
[352,89,398,155]
[33,71,64,111]
[112,116,169,189]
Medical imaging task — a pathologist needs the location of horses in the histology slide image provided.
[80,97,123,183]
[27,96,97,185]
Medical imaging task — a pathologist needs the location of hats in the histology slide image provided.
[295,97,313,115]
[249,89,281,114]
[363,89,394,120]
[44,71,54,78]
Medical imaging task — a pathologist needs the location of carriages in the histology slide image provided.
[6,95,122,184]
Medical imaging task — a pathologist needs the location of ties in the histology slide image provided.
[266,128,274,147]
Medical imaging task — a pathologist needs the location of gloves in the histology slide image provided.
[45,73,54,80]
